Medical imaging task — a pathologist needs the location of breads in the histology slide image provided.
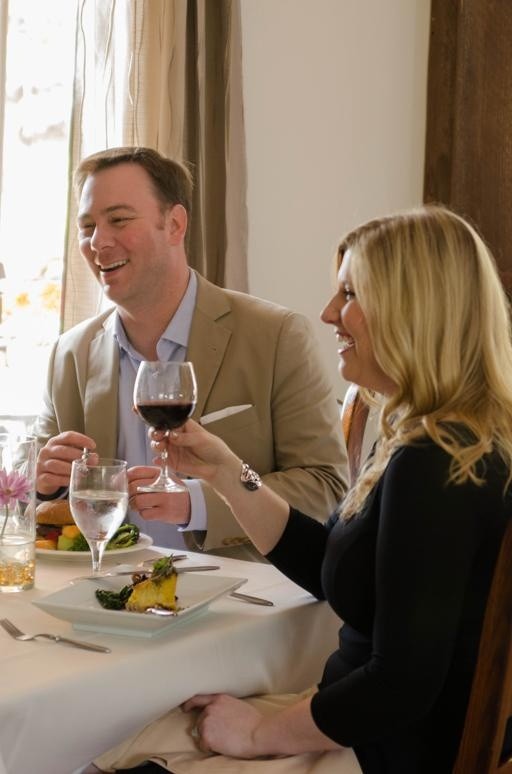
[34,500,76,525]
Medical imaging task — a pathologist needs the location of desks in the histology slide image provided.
[0,508,346,773]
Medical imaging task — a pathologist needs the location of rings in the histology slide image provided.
[192,726,198,738]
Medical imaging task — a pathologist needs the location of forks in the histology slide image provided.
[0,617,111,654]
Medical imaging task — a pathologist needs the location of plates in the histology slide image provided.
[36,532,154,562]
[36,573,250,637]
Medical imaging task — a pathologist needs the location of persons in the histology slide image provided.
[12,146,352,561]
[132,209,512,774]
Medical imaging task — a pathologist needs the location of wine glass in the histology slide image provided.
[134,360,196,493]
[69,458,131,577]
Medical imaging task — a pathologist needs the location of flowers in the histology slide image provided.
[0,468,33,540]
